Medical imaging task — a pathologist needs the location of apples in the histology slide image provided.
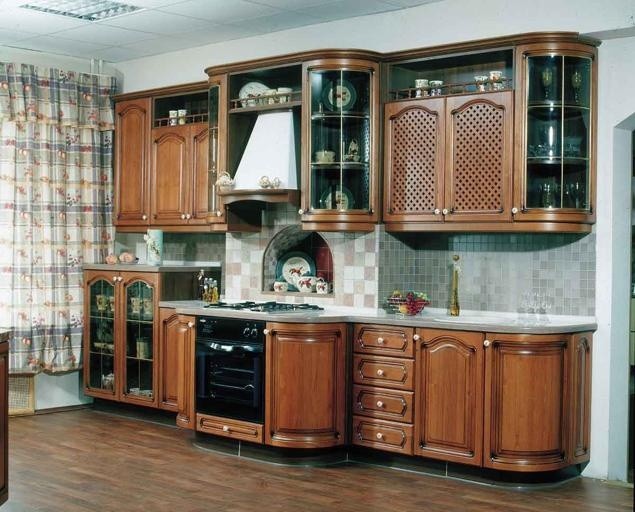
[406,291,414,298]
[392,289,400,298]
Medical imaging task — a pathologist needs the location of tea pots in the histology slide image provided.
[215,171,236,191]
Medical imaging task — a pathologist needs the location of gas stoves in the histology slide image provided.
[203,300,327,315]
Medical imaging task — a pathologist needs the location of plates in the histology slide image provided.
[323,78,357,113]
[319,185,354,209]
[238,80,270,108]
[276,251,316,290]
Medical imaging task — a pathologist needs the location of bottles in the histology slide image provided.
[202,278,218,304]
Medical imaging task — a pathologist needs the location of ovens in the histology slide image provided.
[195,340,263,411]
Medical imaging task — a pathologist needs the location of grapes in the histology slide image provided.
[404,292,430,314]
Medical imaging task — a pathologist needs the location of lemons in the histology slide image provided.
[400,305,409,314]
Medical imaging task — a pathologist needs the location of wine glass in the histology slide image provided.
[541,67,553,101]
[572,73,584,106]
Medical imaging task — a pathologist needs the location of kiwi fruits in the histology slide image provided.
[106,252,136,264]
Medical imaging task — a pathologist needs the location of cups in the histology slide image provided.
[169,110,187,126]
[414,79,444,98]
[95,294,116,311]
[474,71,506,92]
[297,276,330,295]
[265,88,293,106]
[131,297,153,314]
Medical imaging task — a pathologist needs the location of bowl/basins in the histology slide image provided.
[316,151,336,162]
[273,282,289,292]
[343,154,360,162]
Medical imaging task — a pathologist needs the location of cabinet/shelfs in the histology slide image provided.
[143,89,227,227]
[510,44,596,223]
[482,331,595,473]
[111,95,151,227]
[297,58,380,222]
[216,61,302,210]
[261,320,350,447]
[385,47,514,226]
[349,323,419,457]
[414,328,484,467]
[157,307,196,430]
[81,269,221,408]
